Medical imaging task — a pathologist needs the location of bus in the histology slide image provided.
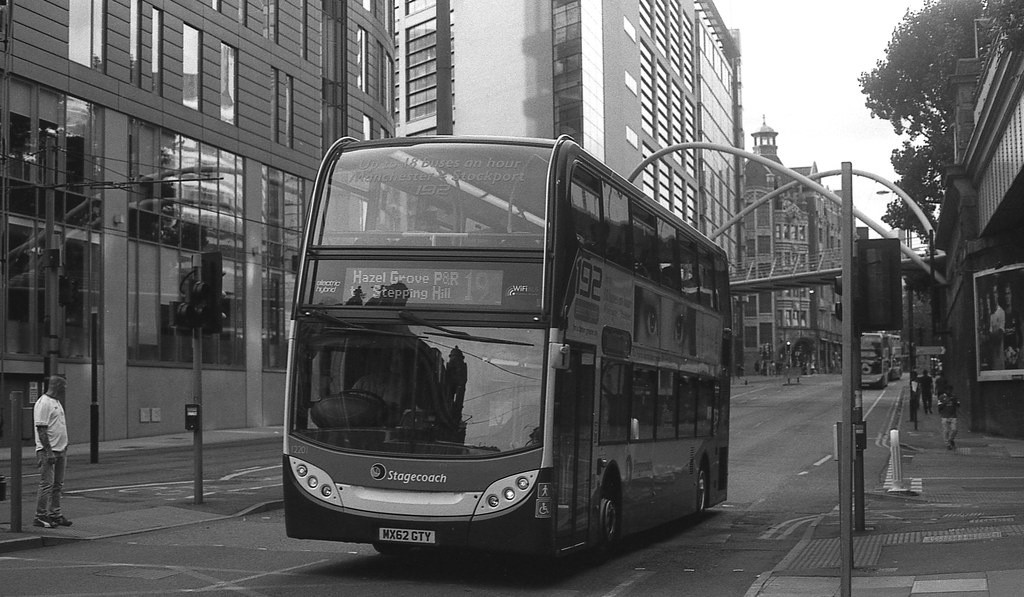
[281,133,733,564]
[859,332,890,389]
[887,334,902,381]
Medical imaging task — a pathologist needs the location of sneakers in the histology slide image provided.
[48,515,73,526]
[33,515,58,527]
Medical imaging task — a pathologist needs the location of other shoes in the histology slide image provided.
[946,444,953,450]
[948,439,955,447]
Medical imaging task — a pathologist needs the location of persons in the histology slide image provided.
[980,284,1020,370]
[34,375,73,527]
[938,385,960,448]
[806,360,818,374]
[918,369,945,413]
[348,345,439,422]
[754,360,761,376]
[770,360,790,375]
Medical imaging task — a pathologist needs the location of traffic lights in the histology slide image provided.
[786,340,791,353]
[170,280,212,331]
